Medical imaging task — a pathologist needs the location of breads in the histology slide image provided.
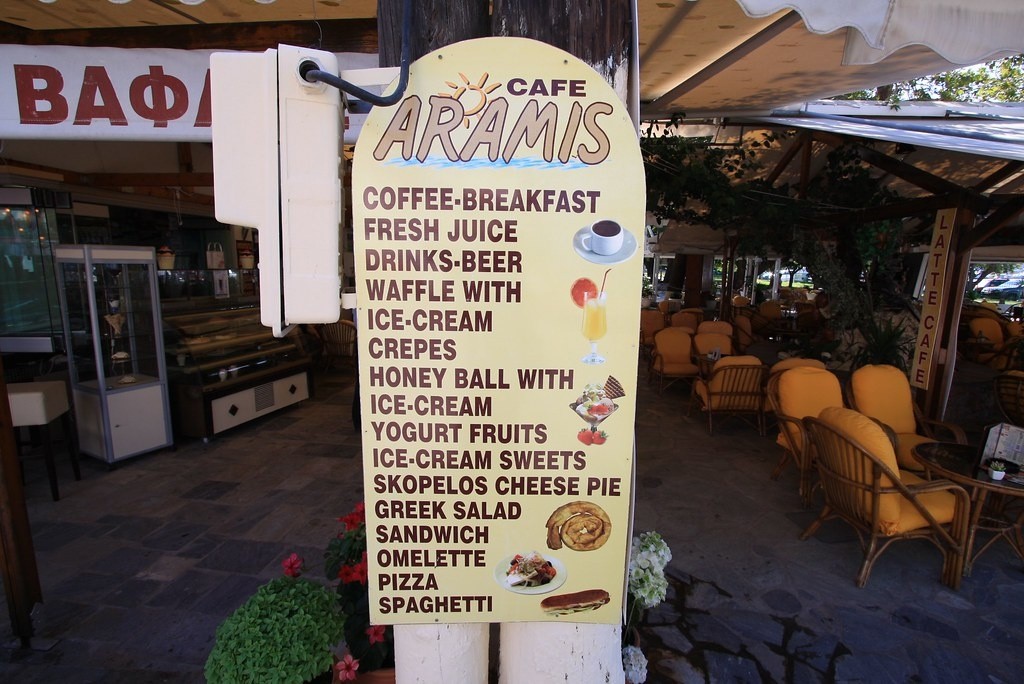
[544,501,612,551]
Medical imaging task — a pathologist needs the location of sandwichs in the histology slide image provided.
[540,589,611,615]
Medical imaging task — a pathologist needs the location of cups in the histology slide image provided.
[579,221,624,256]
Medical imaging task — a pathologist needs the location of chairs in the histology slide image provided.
[640,287,1024,590]
[320,320,358,375]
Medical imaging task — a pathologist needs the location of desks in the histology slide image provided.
[910,442,1024,577]
[699,354,729,369]
[962,341,995,361]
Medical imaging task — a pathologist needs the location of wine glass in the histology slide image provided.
[580,291,609,365]
[569,403,619,433]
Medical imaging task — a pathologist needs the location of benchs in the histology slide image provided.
[7,381,80,501]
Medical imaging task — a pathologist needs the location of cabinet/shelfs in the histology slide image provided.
[0,185,315,472]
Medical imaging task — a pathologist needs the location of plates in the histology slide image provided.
[494,553,568,595]
[574,225,636,264]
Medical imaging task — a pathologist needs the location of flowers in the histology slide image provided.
[281,502,393,682]
[620,531,672,684]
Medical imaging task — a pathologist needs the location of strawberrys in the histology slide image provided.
[577,428,608,446]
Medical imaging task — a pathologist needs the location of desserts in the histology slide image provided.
[574,374,625,427]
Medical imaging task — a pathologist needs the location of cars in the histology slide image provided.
[967,270,1024,301]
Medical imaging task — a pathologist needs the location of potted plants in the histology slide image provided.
[203,574,348,684]
[641,289,652,308]
[358,667,396,684]
[668,291,682,313]
[989,461,1006,480]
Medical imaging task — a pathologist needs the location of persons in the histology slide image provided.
[814,288,825,302]
[778,274,782,288]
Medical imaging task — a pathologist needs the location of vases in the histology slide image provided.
[622,625,641,648]
